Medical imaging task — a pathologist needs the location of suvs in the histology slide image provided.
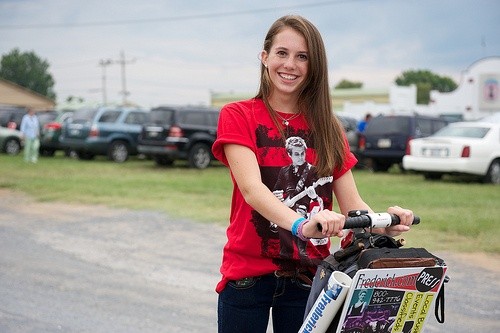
[356,113,449,174]
[136,103,221,170]
[59,107,147,165]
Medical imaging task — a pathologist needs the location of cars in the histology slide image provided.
[0,102,88,164]
[402,121,500,185]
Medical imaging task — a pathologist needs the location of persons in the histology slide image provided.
[5,114,17,130]
[17,108,41,163]
[348,290,368,316]
[268,135,324,266]
[356,113,373,167]
[212,13,414,332]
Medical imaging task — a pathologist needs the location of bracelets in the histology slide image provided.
[291,217,312,242]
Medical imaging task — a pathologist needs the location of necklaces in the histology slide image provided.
[266,98,304,125]
